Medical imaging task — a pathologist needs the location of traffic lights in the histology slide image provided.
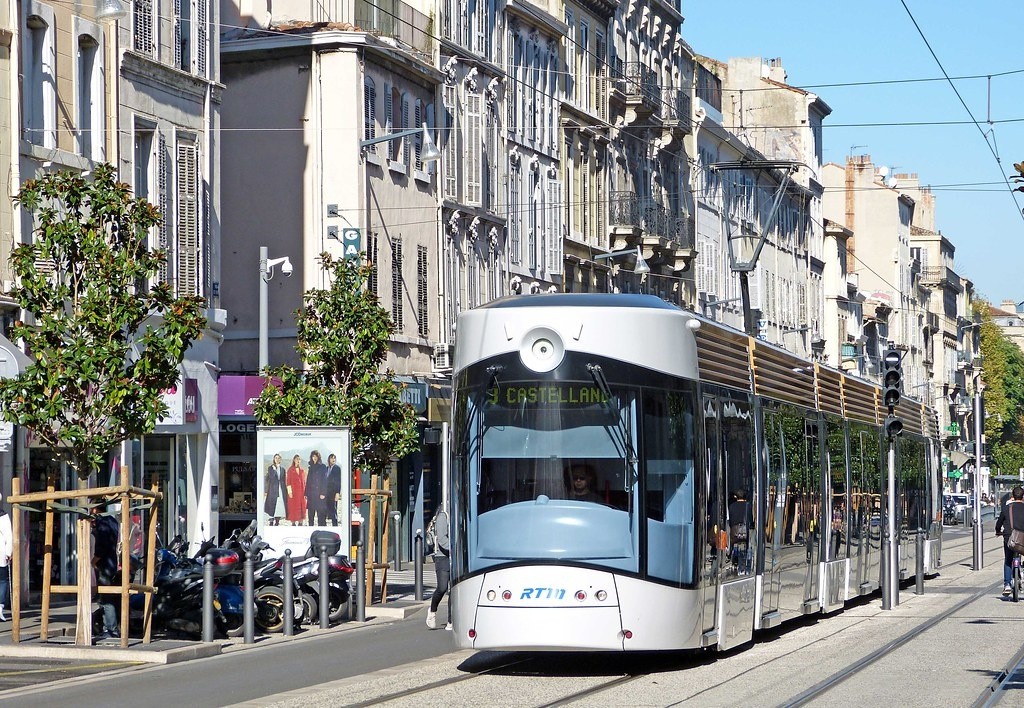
[882,348,904,439]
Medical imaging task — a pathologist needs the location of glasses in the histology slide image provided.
[572,474,586,480]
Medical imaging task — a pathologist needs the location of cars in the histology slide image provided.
[943,492,974,526]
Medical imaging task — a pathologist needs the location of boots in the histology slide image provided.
[0,604,8,622]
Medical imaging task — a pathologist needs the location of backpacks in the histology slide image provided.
[425,509,448,547]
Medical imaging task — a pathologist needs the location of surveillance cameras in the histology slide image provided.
[282,263,294,277]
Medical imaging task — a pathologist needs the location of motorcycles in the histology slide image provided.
[92,519,354,637]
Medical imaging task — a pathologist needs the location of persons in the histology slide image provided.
[710,489,753,584]
[265,454,287,526]
[286,455,306,526]
[0,509,13,622]
[996,487,1024,596]
[565,467,604,503]
[799,501,845,559]
[324,454,341,526]
[980,493,989,503]
[943,495,955,517]
[426,502,452,630]
[89,497,119,637]
[304,450,327,526]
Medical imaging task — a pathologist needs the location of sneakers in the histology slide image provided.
[444,622,453,631]
[426,606,439,629]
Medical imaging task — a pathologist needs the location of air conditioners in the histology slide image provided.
[435,343,456,369]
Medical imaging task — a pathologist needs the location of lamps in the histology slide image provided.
[592,245,650,274]
[703,294,744,316]
[358,122,442,164]
[781,319,821,344]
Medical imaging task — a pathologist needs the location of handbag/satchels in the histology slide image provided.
[1007,528,1024,555]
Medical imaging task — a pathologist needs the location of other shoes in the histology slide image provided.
[95,631,119,639]
[1001,585,1012,596]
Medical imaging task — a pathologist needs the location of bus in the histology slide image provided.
[450,161,944,652]
